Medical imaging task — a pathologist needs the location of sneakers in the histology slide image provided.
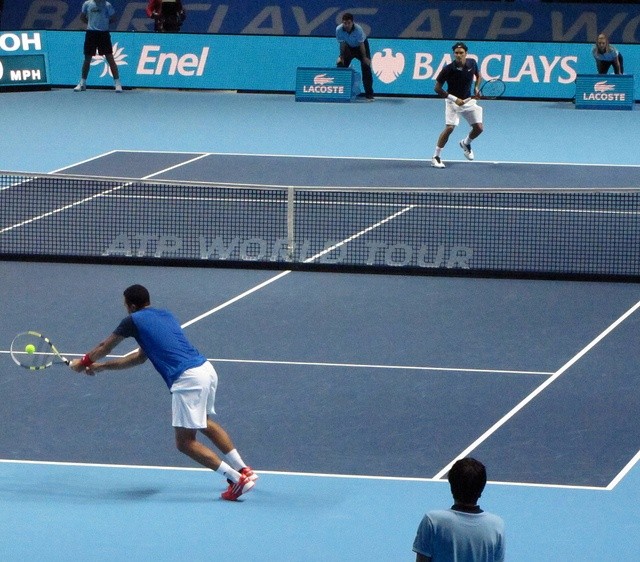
[73,84,86,92]
[221,474,255,500]
[115,85,122,93]
[459,139,474,160]
[366,95,375,101]
[239,465,258,481]
[432,155,445,168]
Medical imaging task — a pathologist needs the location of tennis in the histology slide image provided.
[25,344,35,354]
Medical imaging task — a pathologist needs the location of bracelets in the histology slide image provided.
[80,354,93,367]
[446,90,461,104]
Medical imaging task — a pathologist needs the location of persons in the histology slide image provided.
[411,457,509,562]
[588,33,626,76]
[73,1,124,91]
[430,42,485,168]
[147,0,187,31]
[70,284,259,500]
[335,14,377,98]
